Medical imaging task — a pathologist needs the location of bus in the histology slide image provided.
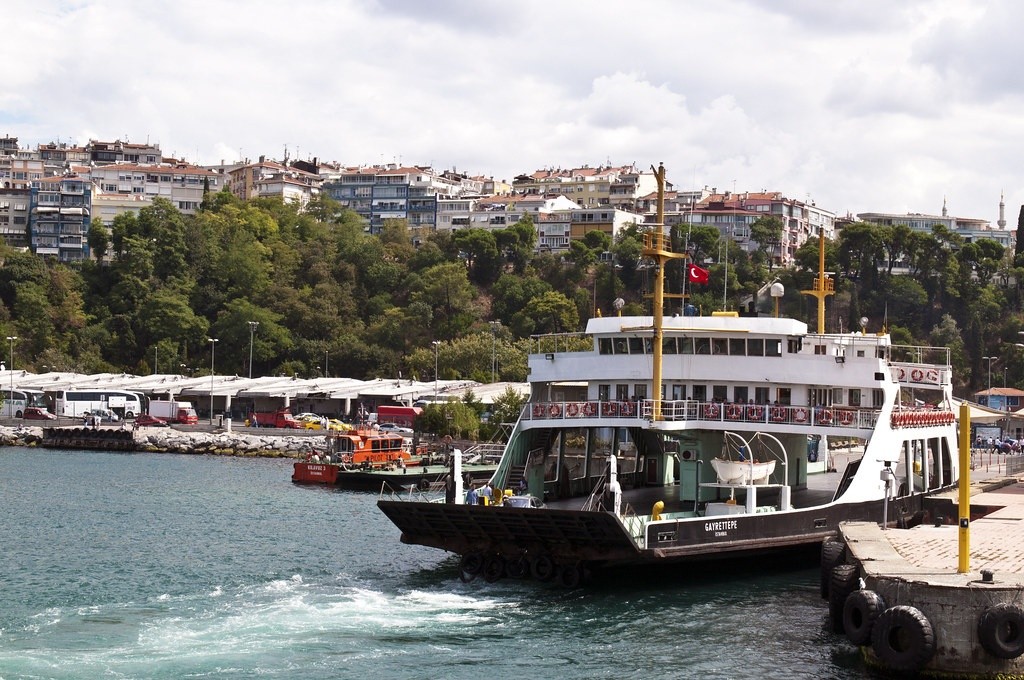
[0,388,57,421]
[57,387,150,420]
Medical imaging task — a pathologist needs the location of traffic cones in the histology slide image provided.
[293,403,376,433]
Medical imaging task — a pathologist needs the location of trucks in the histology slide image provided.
[149,399,199,425]
[378,405,425,433]
[248,406,302,429]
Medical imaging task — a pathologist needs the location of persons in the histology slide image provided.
[662,397,786,422]
[121,421,139,431]
[974,435,1024,455]
[808,450,817,462]
[84,415,102,429]
[466,484,480,505]
[373,422,380,431]
[482,482,492,500]
[685,300,698,316]
[320,415,329,431]
[618,396,650,417]
[17,424,22,431]
[251,413,258,428]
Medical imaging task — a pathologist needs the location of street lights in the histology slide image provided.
[208,338,218,425]
[247,321,260,379]
[6,336,18,416]
[432,341,442,404]
[488,322,500,382]
[1004,367,1008,388]
[982,356,997,407]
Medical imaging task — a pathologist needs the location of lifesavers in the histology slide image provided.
[817,410,832,424]
[897,368,905,379]
[727,406,742,418]
[927,371,938,381]
[458,548,581,589]
[534,402,596,417]
[602,402,616,415]
[641,403,653,415]
[748,406,762,420]
[772,408,786,421]
[343,455,350,463]
[306,453,312,461]
[911,369,924,381]
[705,404,719,418]
[621,402,634,415]
[418,479,431,491]
[443,435,452,444]
[792,408,807,422]
[891,412,955,426]
[839,411,854,424]
[465,474,473,486]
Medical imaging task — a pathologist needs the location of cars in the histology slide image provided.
[132,414,168,428]
[501,495,548,510]
[378,423,414,435]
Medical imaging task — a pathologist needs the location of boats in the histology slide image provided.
[291,402,496,493]
[374,160,962,593]
[709,456,775,486]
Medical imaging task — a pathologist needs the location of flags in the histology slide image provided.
[689,262,709,286]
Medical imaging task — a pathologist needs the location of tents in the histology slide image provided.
[1011,408,1024,418]
[938,395,1019,423]
